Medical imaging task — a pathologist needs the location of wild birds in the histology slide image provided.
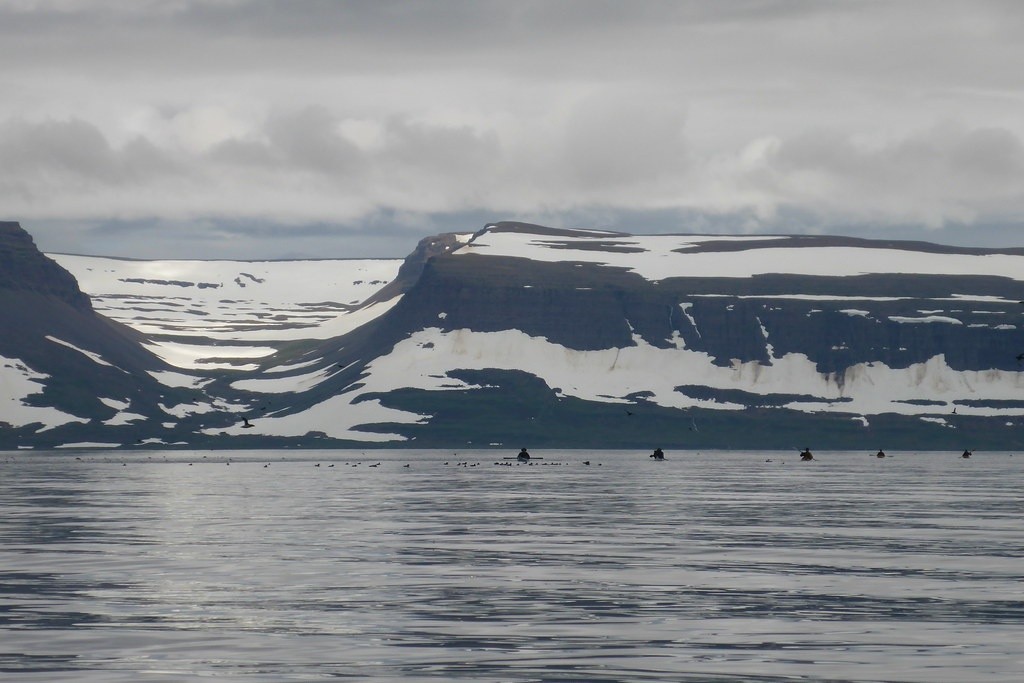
[240,417,255,429]
[444,461,448,465]
[4,453,169,467]
[624,410,635,416]
[264,462,271,467]
[403,463,409,467]
[315,463,320,467]
[203,455,206,458]
[328,464,334,467]
[281,457,285,460]
[952,408,957,414]
[689,416,698,432]
[226,458,232,465]
[362,452,381,467]
[345,462,361,467]
[454,453,456,455]
[188,463,193,466]
[457,461,480,467]
[493,461,603,467]
[766,457,786,465]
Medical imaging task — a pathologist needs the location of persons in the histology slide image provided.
[962,449,972,458]
[877,449,885,458]
[654,448,664,459]
[800,448,813,461]
[516,448,531,461]
